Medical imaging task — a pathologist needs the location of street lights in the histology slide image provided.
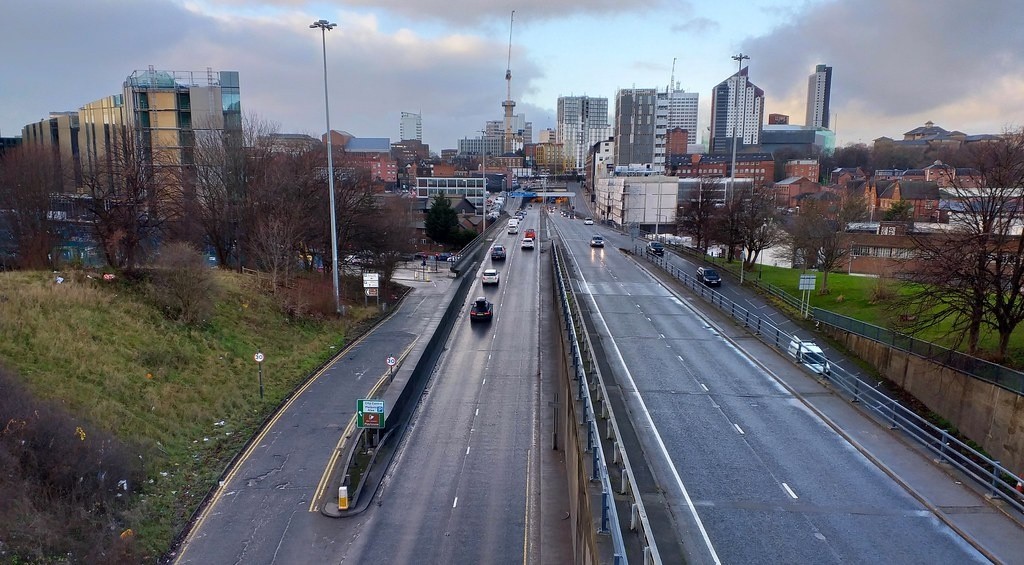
[309,18,341,314]
[730,53,751,203]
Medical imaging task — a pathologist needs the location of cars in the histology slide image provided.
[546,197,576,220]
[584,217,594,225]
[344,247,463,266]
[521,237,535,249]
[646,241,665,256]
[482,268,500,287]
[487,182,538,235]
[470,298,493,322]
[590,235,604,248]
[525,229,536,240]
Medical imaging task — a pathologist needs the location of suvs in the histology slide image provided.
[491,245,507,260]
[788,338,831,381]
[697,266,722,286]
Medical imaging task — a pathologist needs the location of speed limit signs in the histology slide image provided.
[253,351,266,364]
[387,357,396,365]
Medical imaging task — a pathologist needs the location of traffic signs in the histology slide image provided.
[357,399,385,429]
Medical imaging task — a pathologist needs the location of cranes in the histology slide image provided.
[669,57,677,93]
[501,9,515,154]
